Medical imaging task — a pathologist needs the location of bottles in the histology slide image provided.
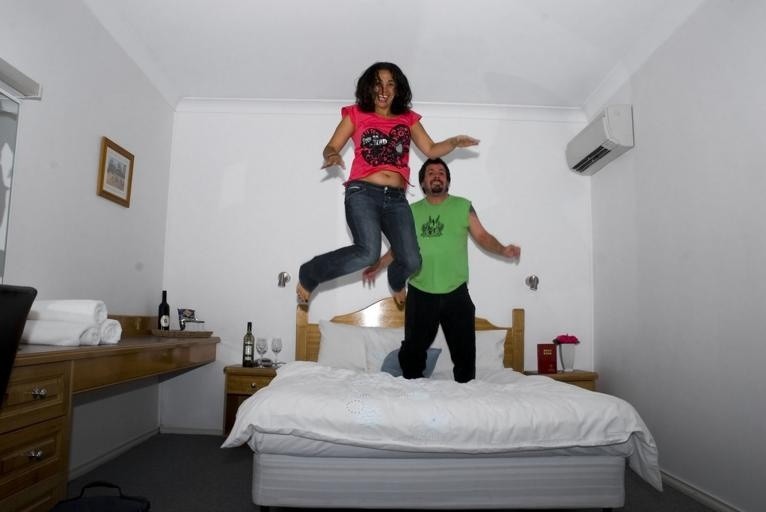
[243,321,255,368]
[158,290,170,330]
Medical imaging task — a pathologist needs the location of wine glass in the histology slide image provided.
[270,337,282,367]
[256,338,268,369]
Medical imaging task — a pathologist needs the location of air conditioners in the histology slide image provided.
[566,104,635,178]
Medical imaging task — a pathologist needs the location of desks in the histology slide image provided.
[2,314,221,509]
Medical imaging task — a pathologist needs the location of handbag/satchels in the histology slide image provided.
[50,480,151,511]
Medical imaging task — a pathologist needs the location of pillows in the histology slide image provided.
[315,319,509,380]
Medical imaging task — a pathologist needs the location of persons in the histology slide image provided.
[296,61,480,303]
[361,156,521,383]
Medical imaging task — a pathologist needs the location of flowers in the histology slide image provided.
[551,335,581,345]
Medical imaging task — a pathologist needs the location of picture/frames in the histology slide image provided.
[97,135,135,210]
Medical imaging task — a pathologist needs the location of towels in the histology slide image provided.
[21,298,122,348]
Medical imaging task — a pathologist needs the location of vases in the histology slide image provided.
[559,343,575,372]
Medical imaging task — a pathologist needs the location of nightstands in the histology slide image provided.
[524,365,598,393]
[223,363,279,444]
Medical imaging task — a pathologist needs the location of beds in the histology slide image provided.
[222,295,633,508]
